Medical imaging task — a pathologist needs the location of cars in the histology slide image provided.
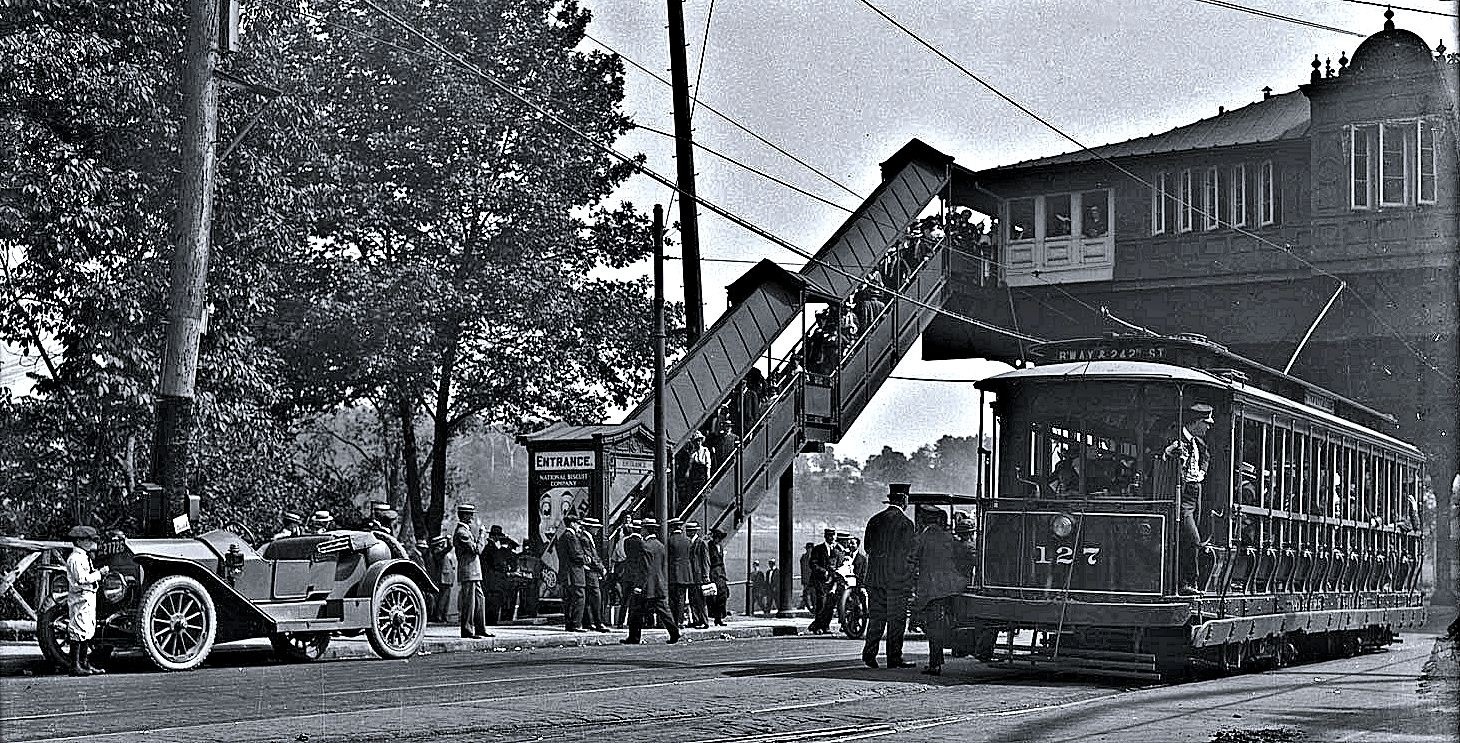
[35,494,441,672]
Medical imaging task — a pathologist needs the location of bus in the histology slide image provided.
[951,307,1430,678]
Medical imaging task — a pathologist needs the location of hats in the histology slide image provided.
[283,510,303,524]
[456,504,479,513]
[486,525,505,536]
[821,528,836,534]
[415,539,429,548]
[430,536,446,545]
[889,483,977,532]
[691,434,705,442]
[68,525,100,541]
[312,511,333,522]
[372,501,391,511]
[834,532,855,541]
[1234,462,1254,478]
[562,514,727,541]
[721,420,734,427]
[1185,403,1215,424]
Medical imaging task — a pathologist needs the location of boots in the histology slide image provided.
[69,643,93,677]
[79,644,105,675]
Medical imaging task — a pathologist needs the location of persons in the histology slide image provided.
[67,526,110,677]
[944,205,991,254]
[280,513,301,535]
[800,529,867,634]
[372,505,399,537]
[1234,461,1422,533]
[1084,206,1105,237]
[711,367,778,470]
[863,484,976,675]
[668,518,728,629]
[557,515,611,634]
[1046,446,1137,492]
[766,558,779,613]
[852,216,946,328]
[415,505,518,638]
[788,302,859,383]
[608,512,681,644]
[751,561,767,614]
[685,430,710,498]
[312,511,333,530]
[1163,404,1214,596]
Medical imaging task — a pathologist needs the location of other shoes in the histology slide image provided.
[1178,585,1205,596]
[887,661,916,669]
[477,632,496,637]
[807,625,832,634]
[564,622,726,632]
[863,657,880,669]
[463,635,481,639]
[921,663,942,675]
[666,635,680,644]
[620,638,639,644]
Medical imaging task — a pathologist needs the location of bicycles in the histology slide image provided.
[837,571,867,639]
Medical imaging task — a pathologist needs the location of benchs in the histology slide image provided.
[264,535,338,561]
